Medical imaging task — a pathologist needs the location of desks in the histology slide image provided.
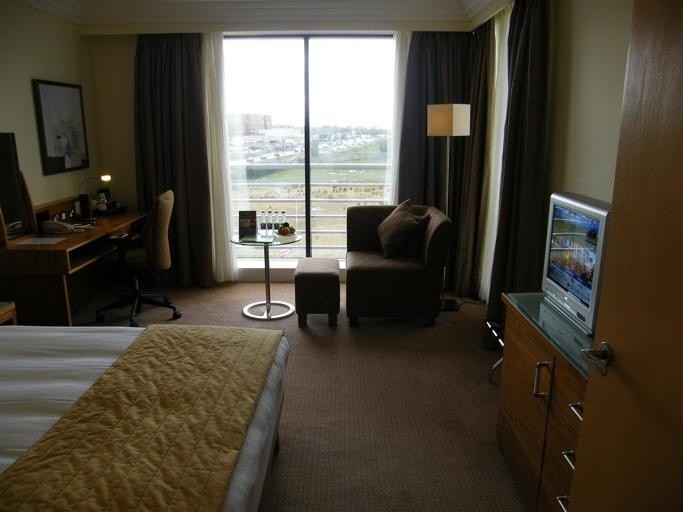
[0,211,153,326]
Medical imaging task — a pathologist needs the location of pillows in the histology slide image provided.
[376,198,430,259]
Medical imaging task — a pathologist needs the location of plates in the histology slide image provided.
[272,230,297,241]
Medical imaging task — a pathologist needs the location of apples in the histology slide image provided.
[279,222,295,235]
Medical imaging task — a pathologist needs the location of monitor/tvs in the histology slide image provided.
[542,191,612,337]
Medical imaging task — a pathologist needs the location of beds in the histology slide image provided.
[1,325,288,512]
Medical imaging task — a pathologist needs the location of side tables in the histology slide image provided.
[229,228,301,321]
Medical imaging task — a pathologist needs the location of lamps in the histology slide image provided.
[425,102,471,312]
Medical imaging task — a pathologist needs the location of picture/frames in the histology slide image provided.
[30,78,89,177]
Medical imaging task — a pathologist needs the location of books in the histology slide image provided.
[239,233,276,244]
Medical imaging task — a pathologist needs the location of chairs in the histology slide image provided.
[344,204,454,328]
[95,190,181,323]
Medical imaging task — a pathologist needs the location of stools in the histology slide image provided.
[293,255,340,328]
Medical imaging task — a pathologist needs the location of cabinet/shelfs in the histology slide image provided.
[497,289,593,512]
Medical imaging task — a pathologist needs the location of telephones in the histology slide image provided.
[41,220,77,233]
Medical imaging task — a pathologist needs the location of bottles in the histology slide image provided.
[259,209,286,237]
[78,194,90,218]
